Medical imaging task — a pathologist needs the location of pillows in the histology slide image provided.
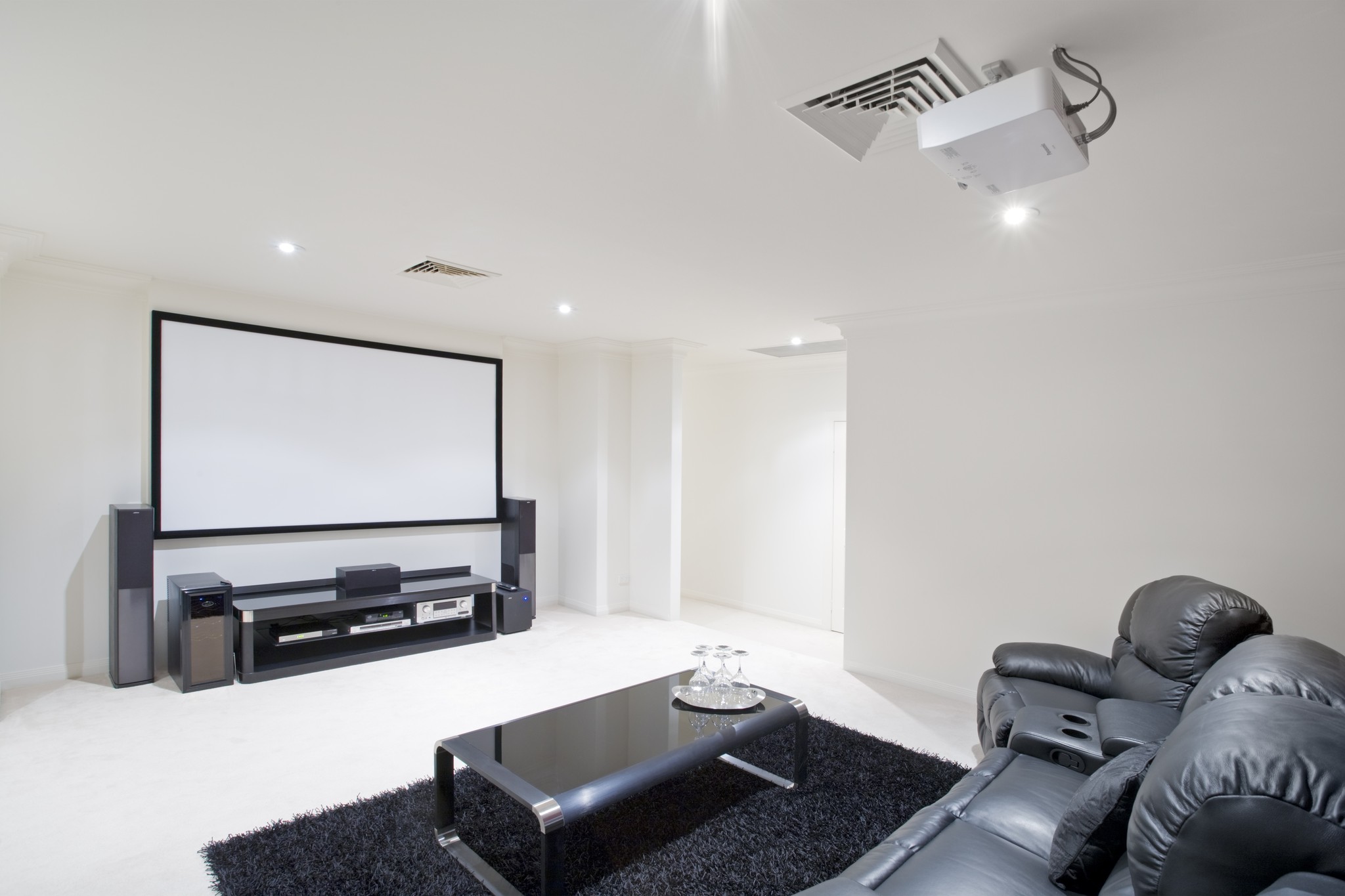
[1047,736,1166,895]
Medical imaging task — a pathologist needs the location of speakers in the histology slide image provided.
[109,503,156,689]
[501,496,537,620]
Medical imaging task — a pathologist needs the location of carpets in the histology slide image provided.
[196,717,973,896]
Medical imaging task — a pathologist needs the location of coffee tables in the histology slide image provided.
[433,666,808,896]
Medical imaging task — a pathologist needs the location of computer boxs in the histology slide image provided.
[167,572,235,694]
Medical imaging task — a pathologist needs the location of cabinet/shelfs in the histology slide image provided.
[232,572,497,685]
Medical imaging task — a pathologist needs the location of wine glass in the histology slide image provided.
[693,644,714,696]
[710,652,733,705]
[713,645,734,696]
[729,650,751,704]
[689,650,710,703]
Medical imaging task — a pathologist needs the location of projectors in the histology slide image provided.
[916,64,1090,202]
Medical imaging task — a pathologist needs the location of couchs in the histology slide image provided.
[977,574,1274,757]
[788,639,1345,896]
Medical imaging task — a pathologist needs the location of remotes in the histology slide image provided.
[496,583,518,592]
